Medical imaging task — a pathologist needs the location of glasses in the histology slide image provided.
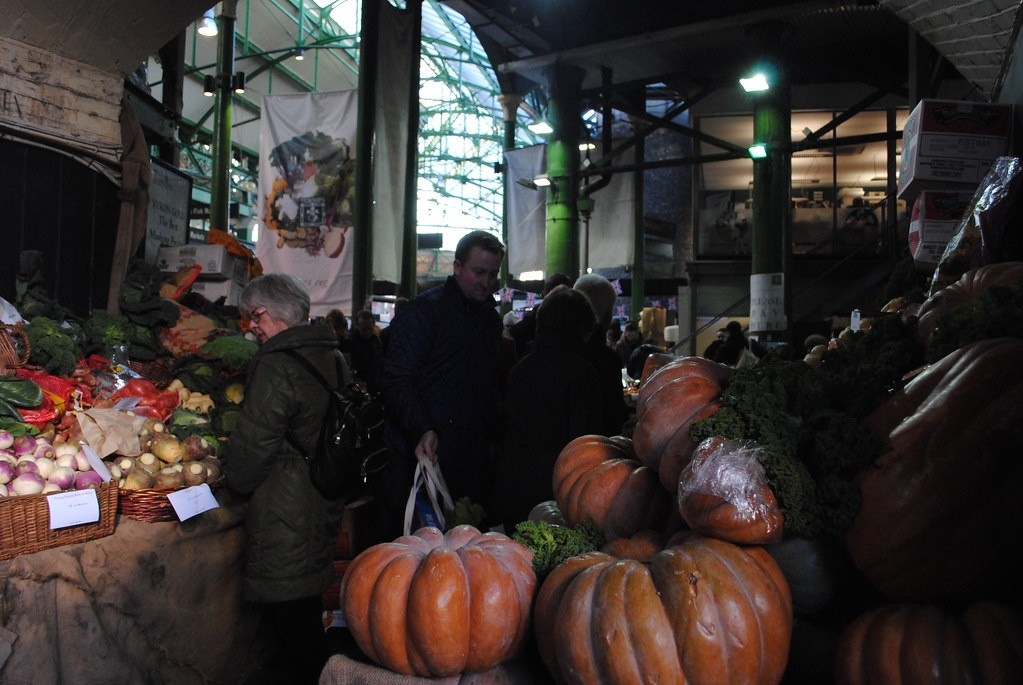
[247,310,267,323]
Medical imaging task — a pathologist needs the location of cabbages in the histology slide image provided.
[166,355,245,455]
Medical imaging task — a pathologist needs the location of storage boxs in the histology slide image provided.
[896,98,1015,273]
[155,242,250,307]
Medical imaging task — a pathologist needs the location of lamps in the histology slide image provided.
[515,177,537,191]
[528,119,557,137]
[802,127,813,138]
[231,71,246,93]
[532,174,555,190]
[198,7,218,37]
[204,74,216,97]
[749,143,768,159]
[296,48,303,60]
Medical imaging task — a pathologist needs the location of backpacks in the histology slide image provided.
[243,348,393,505]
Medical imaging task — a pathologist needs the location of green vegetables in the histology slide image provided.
[0,373,44,436]
[118,259,262,376]
[448,281,1023,575]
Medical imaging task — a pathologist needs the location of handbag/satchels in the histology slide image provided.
[159,263,202,301]
[928,156,1023,299]
[207,228,263,277]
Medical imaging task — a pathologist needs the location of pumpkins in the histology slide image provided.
[912,260,1023,347]
[336,357,1023,685]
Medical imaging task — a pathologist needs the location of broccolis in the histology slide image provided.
[12,299,157,374]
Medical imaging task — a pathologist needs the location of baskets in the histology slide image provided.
[0,320,31,376]
[130,361,170,390]
[119,472,225,523]
[0,478,119,561]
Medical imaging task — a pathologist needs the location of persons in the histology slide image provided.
[326,231,749,555]
[222,273,354,685]
